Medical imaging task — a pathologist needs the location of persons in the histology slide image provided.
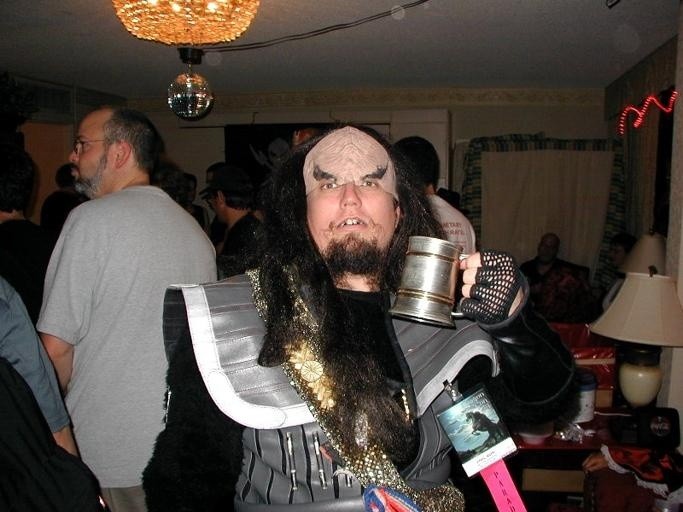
[582,433,683,512]
[0,272,84,457]
[0,349,100,508]
[38,102,223,512]
[40,149,273,283]
[601,236,635,309]
[394,130,477,262]
[518,232,583,316]
[0,147,57,328]
[137,120,580,512]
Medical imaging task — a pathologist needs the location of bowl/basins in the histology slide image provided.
[516,425,553,445]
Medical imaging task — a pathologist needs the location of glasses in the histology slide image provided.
[73,136,120,156]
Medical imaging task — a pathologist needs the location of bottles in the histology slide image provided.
[387,234,485,330]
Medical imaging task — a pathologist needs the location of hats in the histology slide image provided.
[198,162,251,196]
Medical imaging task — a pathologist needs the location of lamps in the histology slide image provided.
[614,231,665,277]
[164,42,214,118]
[586,270,682,412]
[111,0,261,47]
